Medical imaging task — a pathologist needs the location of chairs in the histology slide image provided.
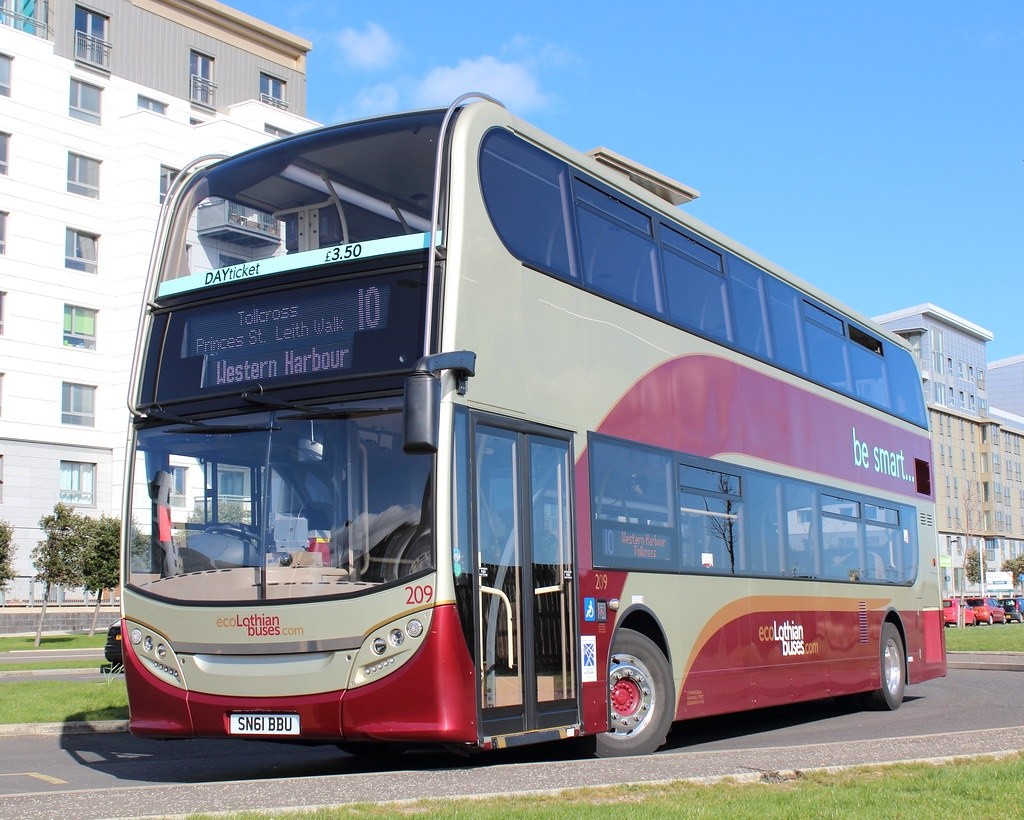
[508,231,764,355]
[839,552,885,580]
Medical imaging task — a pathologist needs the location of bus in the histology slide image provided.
[117,92,949,759]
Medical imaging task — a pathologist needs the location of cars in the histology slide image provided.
[998,597,1024,623]
[965,597,1007,625]
[100,616,124,673]
[943,598,977,629]
